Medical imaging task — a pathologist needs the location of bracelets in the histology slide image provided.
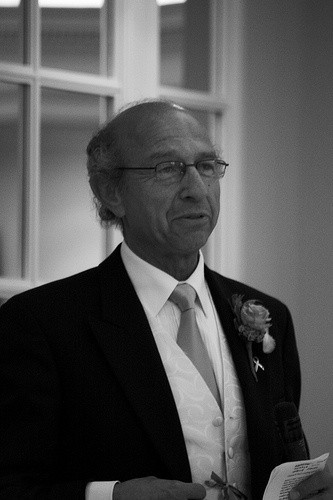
[302,484,330,499]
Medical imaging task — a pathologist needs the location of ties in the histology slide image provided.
[168,282,222,413]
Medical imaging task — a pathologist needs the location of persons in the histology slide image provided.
[0,98,333,500]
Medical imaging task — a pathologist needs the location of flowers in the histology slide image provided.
[236,298,276,381]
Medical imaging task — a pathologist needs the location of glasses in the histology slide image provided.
[114,156,230,179]
